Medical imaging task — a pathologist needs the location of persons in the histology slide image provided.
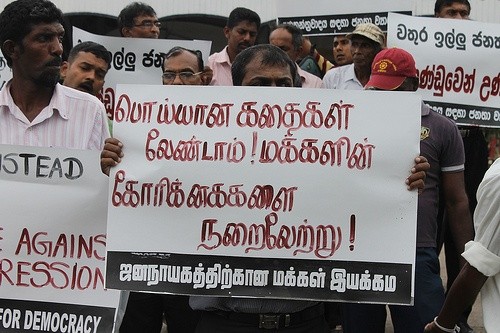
[362,47,474,333]
[434,0,491,257]
[100,45,432,333]
[424,158,500,333]
[59,0,394,332]
[0,0,111,149]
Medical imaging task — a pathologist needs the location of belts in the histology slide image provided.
[203,302,325,330]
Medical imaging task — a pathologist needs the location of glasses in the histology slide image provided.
[161,71,203,85]
[134,21,162,29]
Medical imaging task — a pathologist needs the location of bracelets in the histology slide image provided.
[433,316,460,333]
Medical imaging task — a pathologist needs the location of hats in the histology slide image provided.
[363,47,416,90]
[345,22,385,46]
